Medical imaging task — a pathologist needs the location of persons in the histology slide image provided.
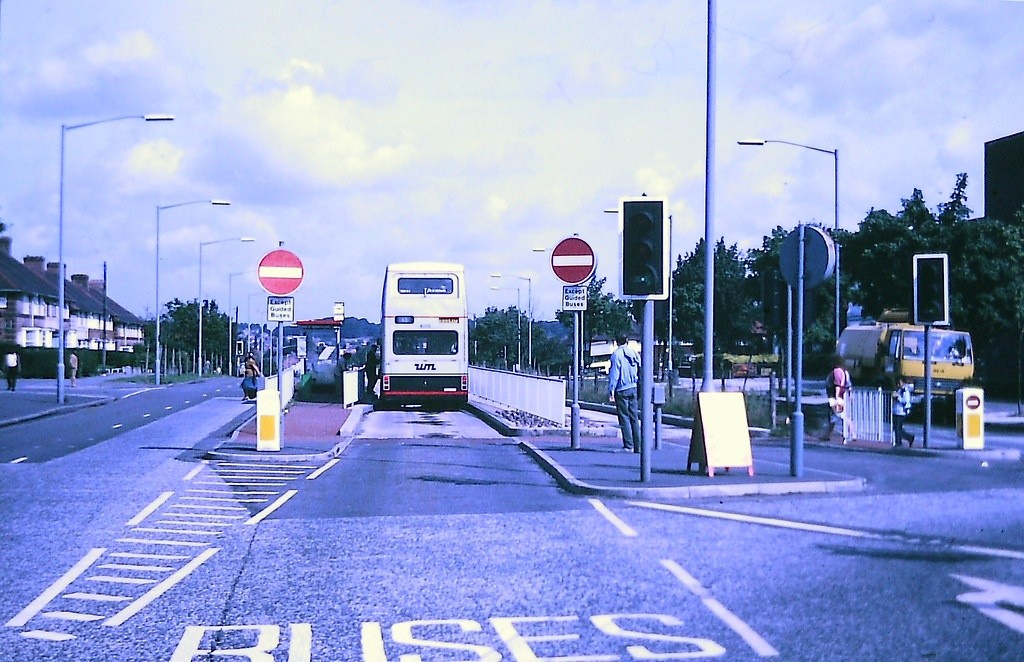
[3,352,18,391]
[240,356,264,401]
[70,349,79,386]
[608,331,640,454]
[317,343,324,353]
[334,342,379,393]
[398,339,409,351]
[819,354,850,441]
[891,376,915,449]
[205,360,210,370]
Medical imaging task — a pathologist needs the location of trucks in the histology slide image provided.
[837,308,975,425]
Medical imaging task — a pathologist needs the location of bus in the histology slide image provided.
[380,261,469,410]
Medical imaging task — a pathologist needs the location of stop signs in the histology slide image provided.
[551,236,597,286]
[966,396,980,410]
[257,249,304,296]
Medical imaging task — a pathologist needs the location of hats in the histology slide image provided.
[371,344,379,348]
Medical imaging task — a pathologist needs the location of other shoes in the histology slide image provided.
[367,391,376,394]
[818,436,830,441]
[621,447,634,453]
[892,442,903,446]
[908,435,914,447]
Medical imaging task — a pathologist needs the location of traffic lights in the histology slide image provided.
[619,196,669,299]
[913,253,949,326]
[235,341,243,356]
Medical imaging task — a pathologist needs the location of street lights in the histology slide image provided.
[736,139,840,347]
[155,198,232,387]
[198,237,256,379]
[57,111,175,406]
[489,273,533,375]
